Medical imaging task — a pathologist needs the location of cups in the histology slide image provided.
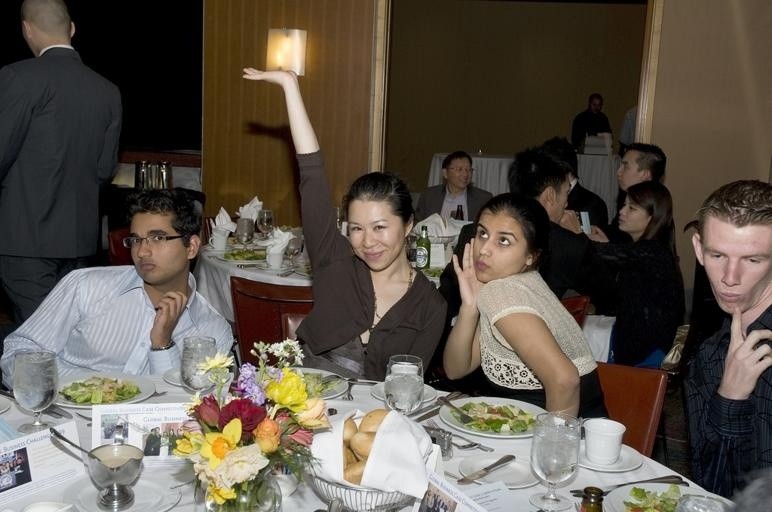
[134,159,172,189]
[583,417,627,464]
[209,230,228,249]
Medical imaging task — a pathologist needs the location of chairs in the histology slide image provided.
[109,228,134,265]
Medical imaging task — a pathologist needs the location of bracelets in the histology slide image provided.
[151,340,175,352]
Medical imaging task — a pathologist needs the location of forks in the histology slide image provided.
[425,418,494,453]
[321,374,376,400]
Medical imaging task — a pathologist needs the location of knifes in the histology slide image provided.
[408,391,470,422]
[0,382,74,419]
[570,475,690,497]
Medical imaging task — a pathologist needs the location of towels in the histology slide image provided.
[210,206,237,233]
[412,214,446,236]
[235,196,264,224]
[258,226,294,253]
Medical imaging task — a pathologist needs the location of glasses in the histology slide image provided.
[121,230,189,248]
[445,165,474,173]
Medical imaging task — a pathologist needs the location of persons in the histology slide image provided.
[438,191,610,419]
[242,66,447,385]
[413,92,686,369]
[1,457,27,476]
[144,427,187,456]
[426,490,449,512]
[683,179,772,499]
[0,0,123,326]
[0,188,235,388]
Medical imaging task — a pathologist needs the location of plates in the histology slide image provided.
[23,472,182,512]
[370,381,550,439]
[0,372,156,414]
[578,440,733,512]
[217,253,267,263]
[163,366,350,399]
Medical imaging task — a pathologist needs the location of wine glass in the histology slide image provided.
[11,336,217,434]
[236,210,304,269]
[384,353,581,512]
[87,444,145,512]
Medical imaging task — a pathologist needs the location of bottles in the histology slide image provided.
[454,203,465,221]
[415,225,431,271]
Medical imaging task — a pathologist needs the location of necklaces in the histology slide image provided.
[375,260,413,320]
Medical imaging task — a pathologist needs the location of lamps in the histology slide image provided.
[266,27,308,76]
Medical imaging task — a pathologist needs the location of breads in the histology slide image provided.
[342,410,389,487]
